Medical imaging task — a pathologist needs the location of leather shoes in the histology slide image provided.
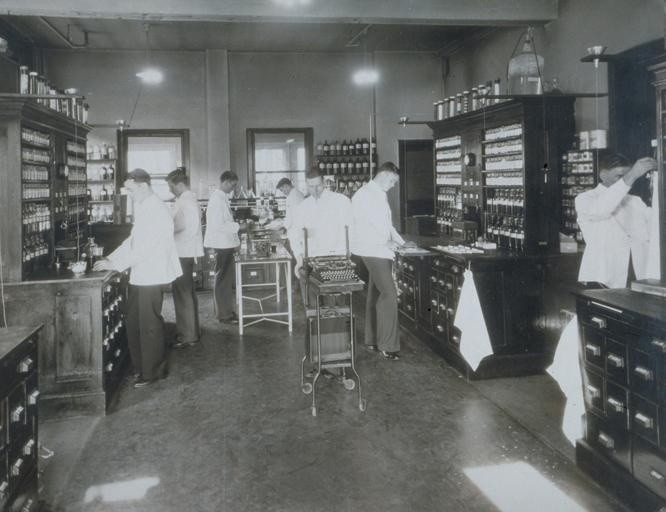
[170,340,199,349]
[133,373,169,388]
[220,311,239,323]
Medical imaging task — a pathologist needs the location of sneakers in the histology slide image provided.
[376,351,402,361]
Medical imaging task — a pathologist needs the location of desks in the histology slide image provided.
[234,242,292,335]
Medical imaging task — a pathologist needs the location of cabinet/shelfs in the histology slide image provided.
[391,92,607,383]
[1,95,134,418]
[0,321,46,512]
[576,39,666,510]
[317,153,378,177]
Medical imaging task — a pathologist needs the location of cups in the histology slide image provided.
[69,262,89,276]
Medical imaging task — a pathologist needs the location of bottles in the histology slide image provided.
[562,131,597,242]
[315,136,379,197]
[433,78,526,254]
[19,127,116,261]
[256,187,280,230]
[19,64,90,127]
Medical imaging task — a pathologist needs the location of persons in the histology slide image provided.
[202,169,249,325]
[91,167,184,388]
[285,166,357,384]
[350,160,419,362]
[574,151,659,289]
[165,168,206,350]
[263,177,304,231]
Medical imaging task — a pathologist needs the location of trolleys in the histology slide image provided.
[300,223,369,418]
